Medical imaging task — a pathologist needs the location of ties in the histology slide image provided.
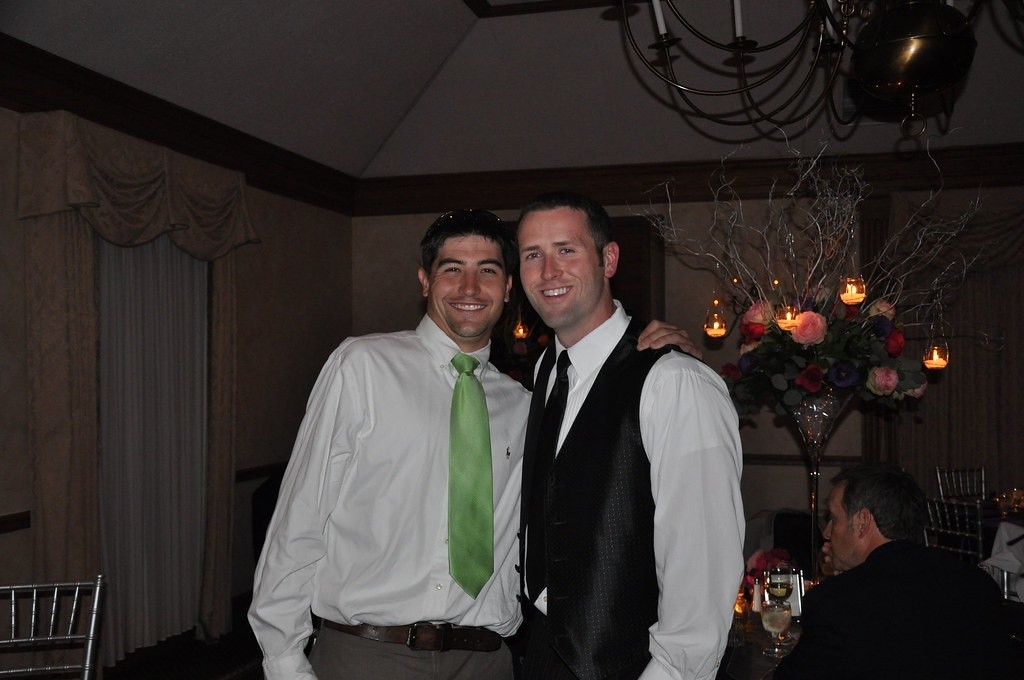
[447,353,495,600]
[526,351,574,601]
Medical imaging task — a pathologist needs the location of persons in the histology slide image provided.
[759,461,1024,680]
[246,209,703,680]
[504,194,746,680]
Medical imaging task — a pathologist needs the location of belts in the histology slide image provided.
[323,618,502,652]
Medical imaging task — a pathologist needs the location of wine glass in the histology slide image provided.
[767,568,793,641]
[761,601,792,657]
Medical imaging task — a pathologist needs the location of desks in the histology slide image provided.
[715,611,804,679]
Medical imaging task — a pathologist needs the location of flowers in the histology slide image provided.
[640,125,1005,578]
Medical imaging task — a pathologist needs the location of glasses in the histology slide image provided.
[432,209,501,232]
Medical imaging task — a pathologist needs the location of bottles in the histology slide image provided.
[728,584,747,647]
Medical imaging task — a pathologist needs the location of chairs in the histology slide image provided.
[0,574,105,680]
